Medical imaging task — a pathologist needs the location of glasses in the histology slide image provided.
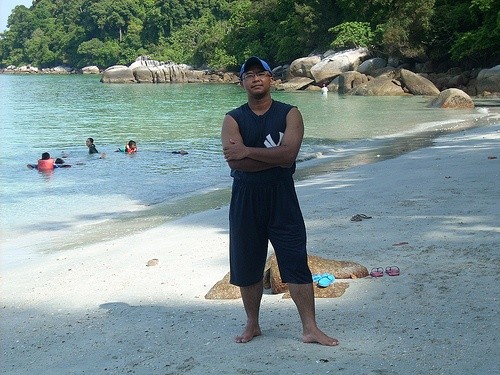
[242,71,271,81]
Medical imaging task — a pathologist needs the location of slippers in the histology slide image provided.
[312,273,321,282]
[318,272,335,287]
[350,214,372,221]
[370,267,383,277]
[385,266,399,276]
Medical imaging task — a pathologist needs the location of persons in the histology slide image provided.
[38,152,53,170]
[86,138,98,154]
[221,56,339,346]
[321,84,328,95]
[114,140,137,154]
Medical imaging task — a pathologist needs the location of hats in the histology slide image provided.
[240,56,272,77]
